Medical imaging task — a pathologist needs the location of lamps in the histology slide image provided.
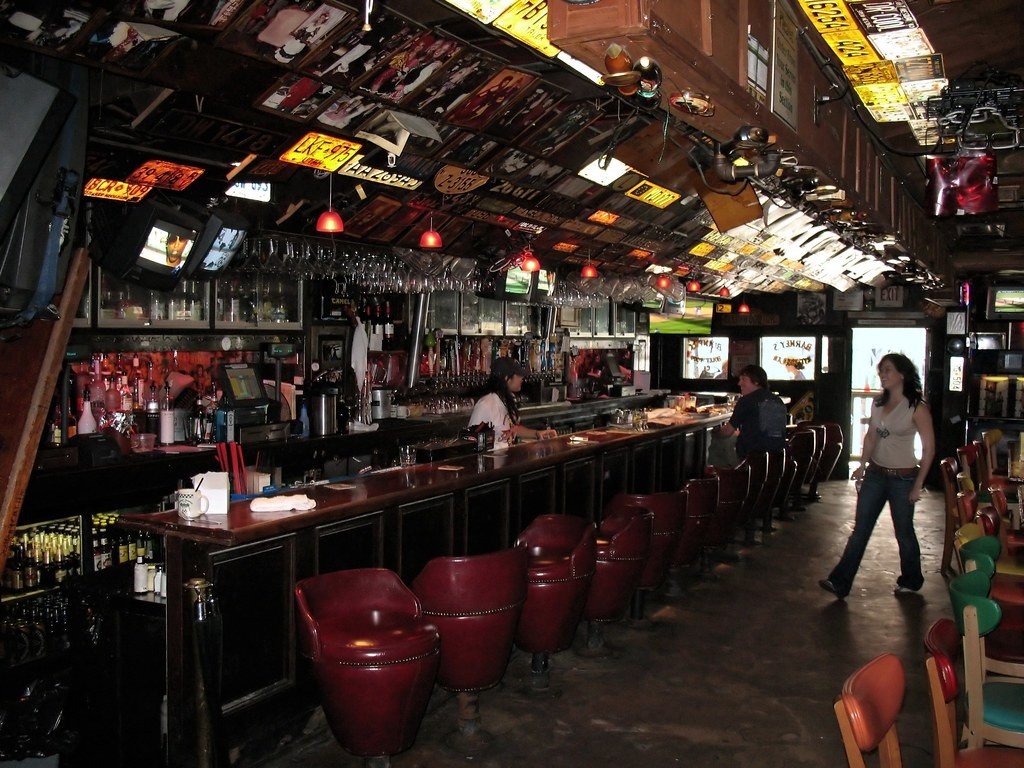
[717,280,732,298]
[655,267,671,289]
[581,245,601,285]
[418,207,444,249]
[520,230,542,278]
[686,269,703,293]
[314,170,345,235]
[737,288,751,316]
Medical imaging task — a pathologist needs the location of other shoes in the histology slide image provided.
[820,578,843,599]
[892,584,919,596]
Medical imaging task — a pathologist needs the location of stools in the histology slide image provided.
[291,417,846,760]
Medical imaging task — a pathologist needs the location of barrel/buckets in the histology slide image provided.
[372,387,395,419]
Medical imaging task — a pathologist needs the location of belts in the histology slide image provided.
[885,469,913,476]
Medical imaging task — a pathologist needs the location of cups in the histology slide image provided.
[178,489,209,521]
[399,445,416,465]
[390,405,410,419]
[409,394,473,414]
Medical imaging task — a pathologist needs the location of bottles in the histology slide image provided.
[0,512,169,759]
[49,347,233,449]
[100,270,289,324]
[359,299,395,342]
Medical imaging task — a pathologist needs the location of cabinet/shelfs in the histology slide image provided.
[0,487,169,702]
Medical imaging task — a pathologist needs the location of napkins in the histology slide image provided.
[246,491,321,516]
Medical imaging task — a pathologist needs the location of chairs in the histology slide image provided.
[829,651,911,768]
[922,427,1024,768]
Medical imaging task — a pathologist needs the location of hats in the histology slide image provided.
[490,356,528,377]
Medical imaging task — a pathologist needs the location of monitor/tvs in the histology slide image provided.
[117,198,203,280]
[605,356,621,378]
[646,297,715,337]
[486,257,533,302]
[533,267,557,295]
[218,362,268,408]
[985,286,1024,321]
[183,201,248,276]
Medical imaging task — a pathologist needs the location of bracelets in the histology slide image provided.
[535,430,539,440]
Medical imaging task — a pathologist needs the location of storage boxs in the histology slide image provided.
[191,470,232,516]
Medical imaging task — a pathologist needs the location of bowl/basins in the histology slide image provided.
[130,433,156,453]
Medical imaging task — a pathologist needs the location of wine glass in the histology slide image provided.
[549,281,610,308]
[237,239,499,296]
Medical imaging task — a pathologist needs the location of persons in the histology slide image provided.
[244,0,278,34]
[466,356,557,452]
[370,35,457,104]
[497,87,551,143]
[165,232,189,271]
[714,360,728,379]
[275,10,331,65]
[449,75,521,127]
[276,76,322,114]
[321,15,413,90]
[317,93,378,130]
[707,365,787,467]
[391,118,564,188]
[419,55,492,113]
[785,358,806,380]
[818,354,934,599]
